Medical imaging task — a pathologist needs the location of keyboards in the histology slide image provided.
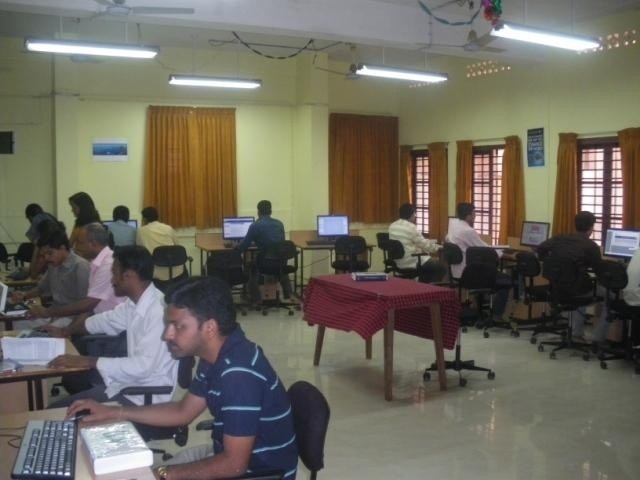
[11,419,78,480]
[28,330,51,338]
[307,240,336,245]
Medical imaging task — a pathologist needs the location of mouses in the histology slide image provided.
[65,409,90,420]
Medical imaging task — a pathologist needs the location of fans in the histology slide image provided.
[419,26,507,54]
[60,1,195,24]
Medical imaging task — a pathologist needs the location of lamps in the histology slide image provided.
[350,11,450,85]
[490,0,602,54]
[23,19,161,59]
[167,31,266,89]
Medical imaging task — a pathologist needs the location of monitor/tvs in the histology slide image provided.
[103,220,137,228]
[317,215,349,240]
[603,228,640,263]
[222,216,255,243]
[447,216,460,233]
[520,221,550,248]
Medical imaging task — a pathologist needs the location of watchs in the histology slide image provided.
[156,466,168,479]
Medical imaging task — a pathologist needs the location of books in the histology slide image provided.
[352,272,387,282]
[79,420,154,476]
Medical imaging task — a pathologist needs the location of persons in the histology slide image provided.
[10,193,185,329]
[389,202,446,284]
[621,244,640,349]
[66,275,300,479]
[235,200,292,302]
[536,211,610,343]
[445,203,511,329]
[34,246,180,410]
